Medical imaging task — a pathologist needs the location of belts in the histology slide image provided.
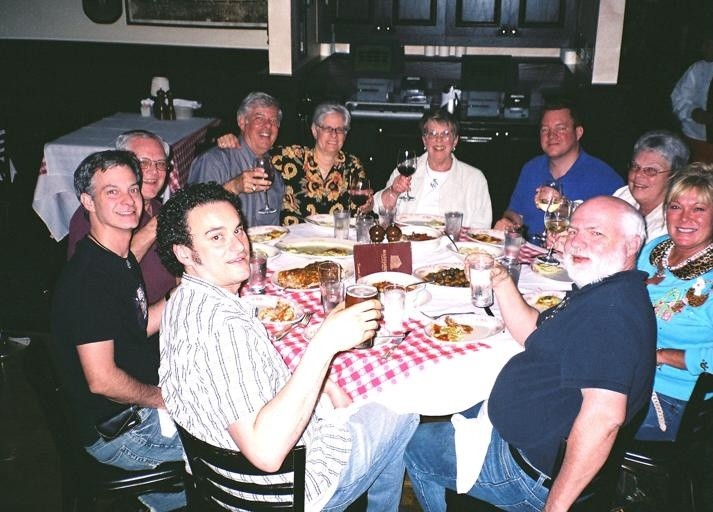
[508,443,554,490]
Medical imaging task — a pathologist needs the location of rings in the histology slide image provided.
[222,143,225,145]
[253,186,256,192]
[396,183,399,187]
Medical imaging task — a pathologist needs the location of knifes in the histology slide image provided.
[381,331,411,361]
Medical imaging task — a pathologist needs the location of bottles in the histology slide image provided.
[153,88,177,122]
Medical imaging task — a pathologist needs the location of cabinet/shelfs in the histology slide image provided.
[447,0,599,49]
[318,0,447,46]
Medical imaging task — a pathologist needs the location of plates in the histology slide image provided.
[240,213,564,344]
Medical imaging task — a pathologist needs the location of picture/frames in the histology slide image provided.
[124,0,268,30]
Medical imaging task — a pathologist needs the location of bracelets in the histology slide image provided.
[656,348,661,369]
[389,186,398,200]
[165,288,173,301]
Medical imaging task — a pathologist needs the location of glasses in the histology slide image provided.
[626,160,672,177]
[314,122,346,134]
[139,161,170,171]
[539,295,571,324]
[424,130,452,140]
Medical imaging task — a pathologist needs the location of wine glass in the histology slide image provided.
[531,180,564,265]
[252,156,278,214]
[397,148,417,200]
[351,177,368,217]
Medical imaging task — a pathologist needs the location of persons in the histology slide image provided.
[158,182,421,512]
[186,91,287,228]
[44,150,187,512]
[543,162,713,442]
[669,33,713,163]
[66,130,181,305]
[404,194,659,512]
[534,129,692,244]
[217,100,375,226]
[373,107,493,228]
[493,97,626,248]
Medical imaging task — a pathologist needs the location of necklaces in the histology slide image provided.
[662,242,713,271]
[545,297,568,320]
[89,232,132,270]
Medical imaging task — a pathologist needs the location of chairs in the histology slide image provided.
[0,118,11,187]
[23,337,186,512]
[445,396,651,512]
[174,419,306,512]
[621,372,713,512]
